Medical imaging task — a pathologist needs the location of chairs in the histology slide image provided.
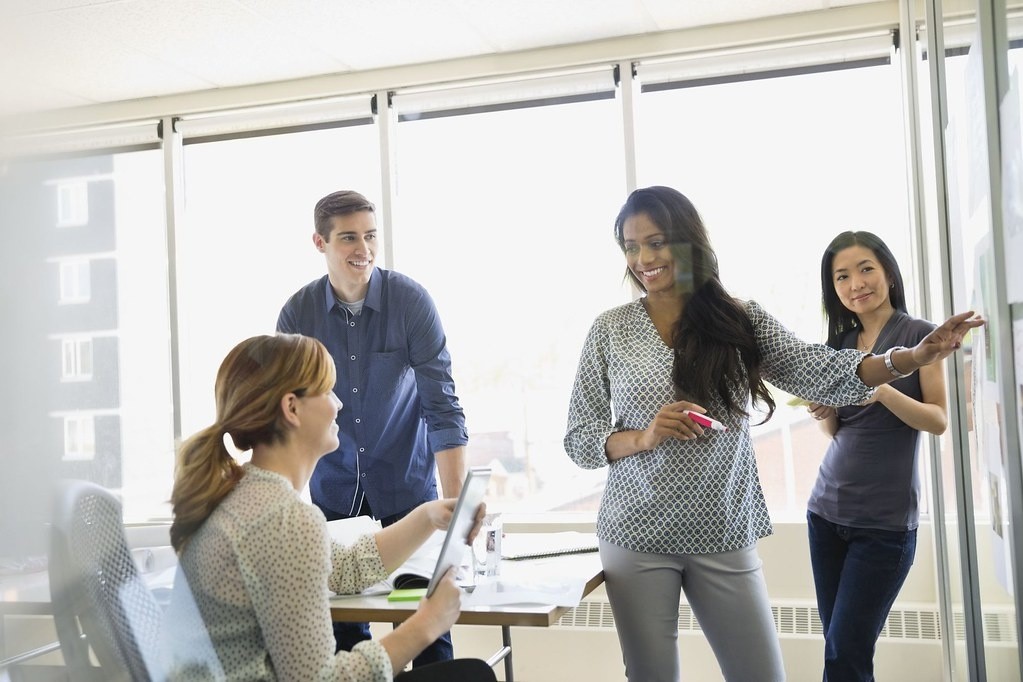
[47,476,174,682]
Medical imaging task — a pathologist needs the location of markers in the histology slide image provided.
[682,409,730,434]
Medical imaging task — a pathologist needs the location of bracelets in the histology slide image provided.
[884,345,914,380]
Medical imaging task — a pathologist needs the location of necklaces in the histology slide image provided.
[859,332,879,350]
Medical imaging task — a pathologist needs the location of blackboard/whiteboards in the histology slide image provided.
[955,0,1023,598]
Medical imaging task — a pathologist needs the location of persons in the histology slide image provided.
[274,191,470,677]
[807,230,949,682]
[562,185,989,682]
[170,331,499,682]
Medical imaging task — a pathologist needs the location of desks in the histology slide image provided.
[330,534,604,682]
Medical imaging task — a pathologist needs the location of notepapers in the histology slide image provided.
[388,587,428,602]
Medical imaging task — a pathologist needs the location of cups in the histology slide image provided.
[473,511,503,584]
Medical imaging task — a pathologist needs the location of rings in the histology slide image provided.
[819,416,824,420]
